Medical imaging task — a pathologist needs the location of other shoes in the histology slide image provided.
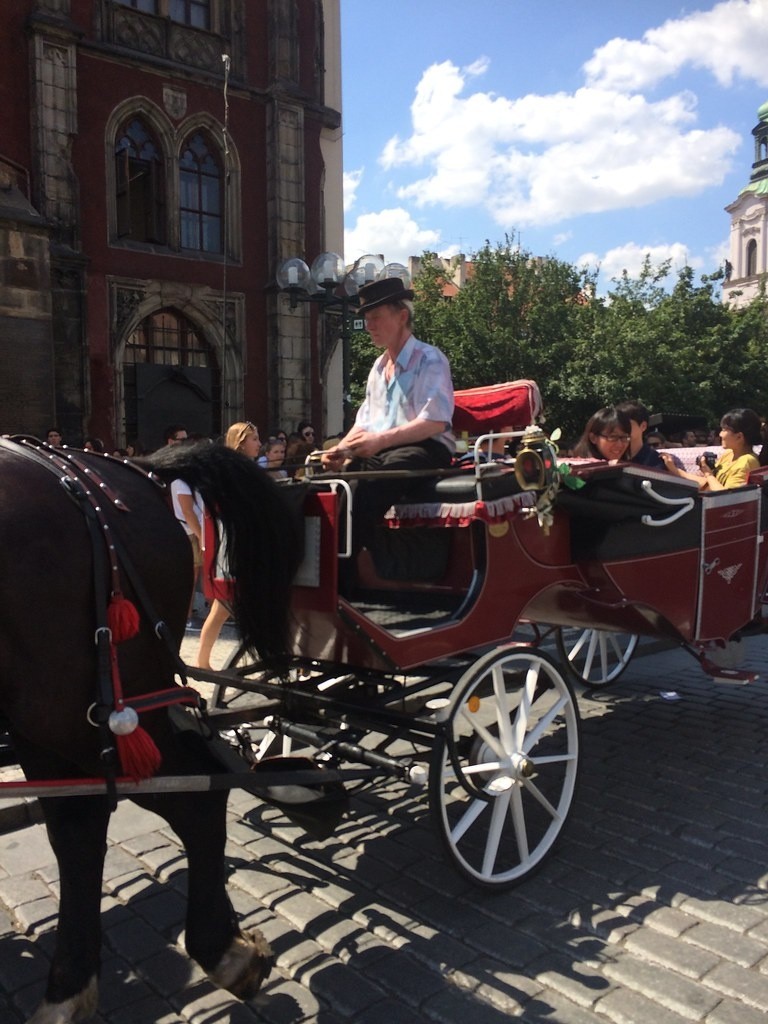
[224,617,236,624]
[185,618,201,631]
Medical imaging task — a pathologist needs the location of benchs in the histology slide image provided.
[557,444,768,564]
[304,378,556,529]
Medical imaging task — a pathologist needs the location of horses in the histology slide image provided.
[0,437,305,1023]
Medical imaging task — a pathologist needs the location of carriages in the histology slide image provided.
[0,377,762,1001]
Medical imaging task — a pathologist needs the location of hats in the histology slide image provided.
[357,278,414,316]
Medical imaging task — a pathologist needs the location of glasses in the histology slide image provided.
[278,437,287,442]
[239,421,251,440]
[170,437,189,442]
[597,432,631,442]
[304,432,316,437]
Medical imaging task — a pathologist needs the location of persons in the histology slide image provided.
[644,431,665,449]
[706,426,722,446]
[459,422,514,463]
[616,399,686,477]
[681,430,697,447]
[196,421,262,672]
[47,428,64,446]
[321,277,455,605]
[85,438,104,453]
[658,407,768,492]
[573,407,632,462]
[111,421,349,631]
